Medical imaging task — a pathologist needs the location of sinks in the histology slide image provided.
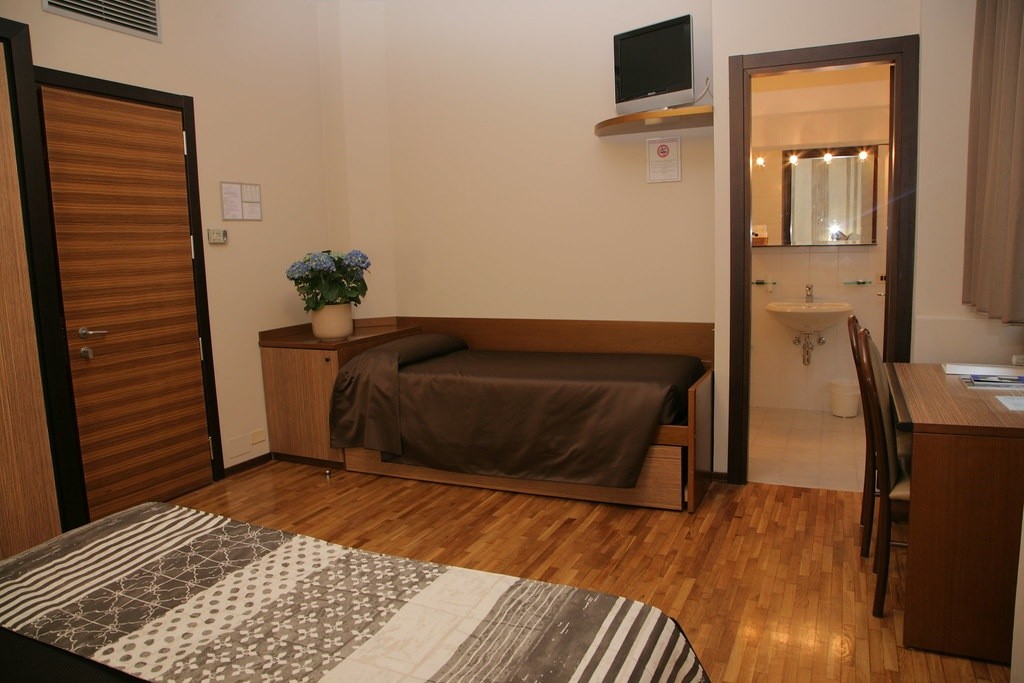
[765,301,853,334]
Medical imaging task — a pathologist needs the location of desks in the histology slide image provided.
[885,360,1024,666]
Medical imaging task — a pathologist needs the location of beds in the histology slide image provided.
[0,497,717,683]
[255,312,715,515]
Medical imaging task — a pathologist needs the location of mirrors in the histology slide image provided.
[747,142,878,248]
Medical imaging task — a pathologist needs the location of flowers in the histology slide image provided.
[280,249,374,313]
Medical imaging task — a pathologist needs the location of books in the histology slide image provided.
[962,375,1024,389]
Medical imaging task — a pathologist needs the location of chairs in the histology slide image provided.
[847,310,914,623]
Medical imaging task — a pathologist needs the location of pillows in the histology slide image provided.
[364,331,471,373]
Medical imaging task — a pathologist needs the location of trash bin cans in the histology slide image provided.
[830,379,861,417]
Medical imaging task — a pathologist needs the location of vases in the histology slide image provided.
[306,296,362,346]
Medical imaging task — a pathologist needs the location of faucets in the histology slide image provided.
[805,284,813,302]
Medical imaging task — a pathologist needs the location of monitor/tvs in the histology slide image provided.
[615,14,693,115]
